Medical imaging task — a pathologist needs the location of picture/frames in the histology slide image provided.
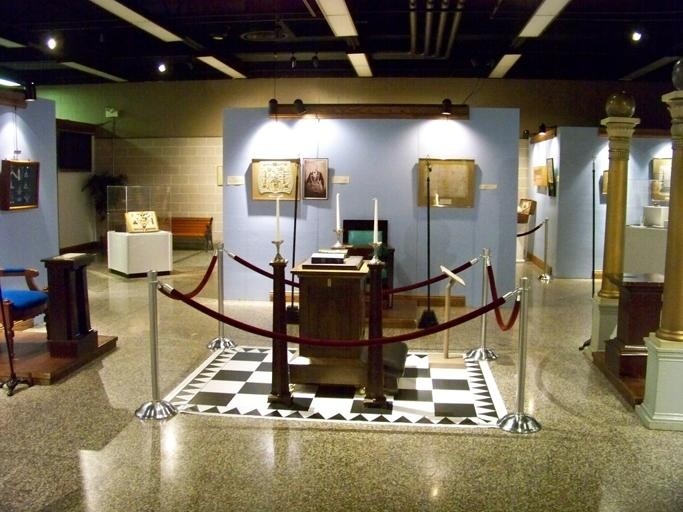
[419,158,476,208]
[302,158,329,199]
[250,158,301,201]
[649,157,673,205]
[546,157,554,184]
[602,170,608,195]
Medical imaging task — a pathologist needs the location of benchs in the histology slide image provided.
[157,217,214,253]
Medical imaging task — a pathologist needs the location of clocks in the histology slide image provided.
[0,160,40,210]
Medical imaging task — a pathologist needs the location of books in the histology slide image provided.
[310,253,344,265]
[317,249,349,258]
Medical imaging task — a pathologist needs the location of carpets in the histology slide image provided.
[162,346,507,433]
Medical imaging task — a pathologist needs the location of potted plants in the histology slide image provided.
[82,172,130,249]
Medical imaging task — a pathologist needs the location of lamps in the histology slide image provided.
[24,84,36,102]
[269,99,470,120]
[522,122,557,144]
[270,44,320,70]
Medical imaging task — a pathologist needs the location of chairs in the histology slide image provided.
[0,267,49,377]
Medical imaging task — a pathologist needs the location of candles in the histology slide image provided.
[335,193,340,230]
[372,198,378,244]
[275,197,280,241]
